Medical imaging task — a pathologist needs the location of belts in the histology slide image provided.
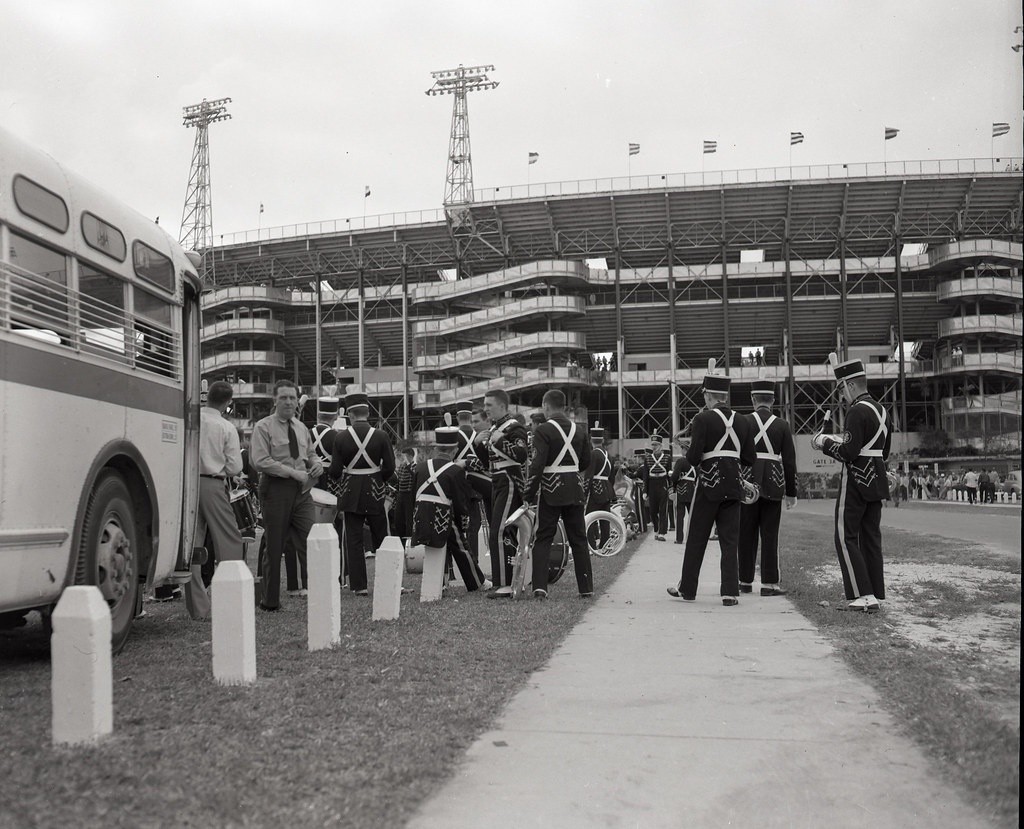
[200,474,224,481]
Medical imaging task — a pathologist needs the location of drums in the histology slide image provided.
[229,488,258,533]
[521,519,570,586]
[404,538,425,574]
[309,487,339,525]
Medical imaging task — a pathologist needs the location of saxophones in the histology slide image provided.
[673,418,761,505]
[810,409,899,495]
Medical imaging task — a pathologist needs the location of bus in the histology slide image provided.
[0,127,202,655]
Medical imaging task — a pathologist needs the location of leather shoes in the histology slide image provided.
[667,588,695,600]
[738,582,788,596]
[836,594,880,612]
[722,596,738,606]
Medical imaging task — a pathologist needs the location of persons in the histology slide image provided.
[134,375,758,622]
[816,358,892,611]
[738,381,798,597]
[749,349,761,366]
[883,467,999,507]
[567,356,607,377]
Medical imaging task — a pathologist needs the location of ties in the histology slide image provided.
[287,420,300,460]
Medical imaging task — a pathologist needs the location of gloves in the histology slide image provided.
[815,434,844,447]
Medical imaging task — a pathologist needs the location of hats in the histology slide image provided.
[590,420,604,439]
[827,352,866,388]
[633,448,670,456]
[200,380,210,403]
[435,401,474,446]
[651,435,663,445]
[941,473,945,477]
[701,358,775,395]
[318,385,370,414]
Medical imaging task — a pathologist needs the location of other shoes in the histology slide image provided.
[149,588,182,602]
[478,580,492,590]
[260,602,283,612]
[488,588,594,600]
[655,535,720,544]
[288,589,415,598]
[365,551,376,558]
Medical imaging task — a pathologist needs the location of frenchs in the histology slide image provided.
[584,462,638,558]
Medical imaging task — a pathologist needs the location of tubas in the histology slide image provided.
[503,505,535,601]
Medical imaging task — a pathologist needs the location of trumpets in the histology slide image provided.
[477,500,491,557]
